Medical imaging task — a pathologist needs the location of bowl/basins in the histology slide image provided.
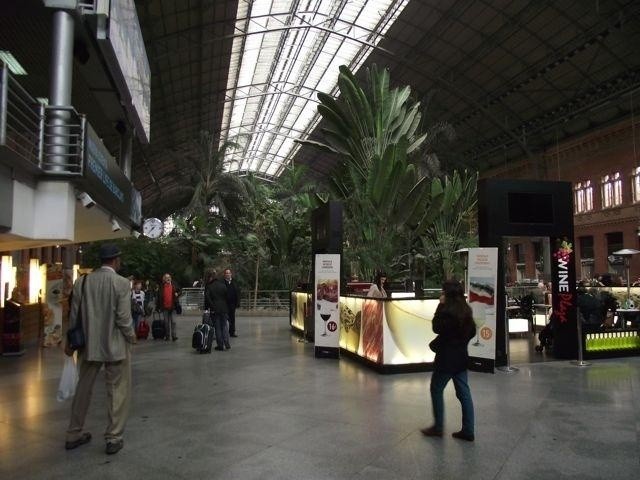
[384,300,441,360]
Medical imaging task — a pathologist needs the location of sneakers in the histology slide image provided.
[165,334,238,352]
[420,426,443,438]
[65,432,93,450]
[105,439,124,455]
[452,432,475,442]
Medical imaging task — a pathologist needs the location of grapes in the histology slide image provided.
[551,240,573,262]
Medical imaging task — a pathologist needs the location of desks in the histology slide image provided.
[607,309,640,332]
[506,299,553,336]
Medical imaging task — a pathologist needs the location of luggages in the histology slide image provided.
[137,320,149,339]
[151,319,165,339]
[192,324,214,354]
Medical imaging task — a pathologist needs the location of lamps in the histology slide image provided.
[111,219,121,232]
[80,192,96,210]
[131,229,141,240]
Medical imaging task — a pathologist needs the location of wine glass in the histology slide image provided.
[320,313,331,337]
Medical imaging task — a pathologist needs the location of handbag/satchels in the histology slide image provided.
[176,297,183,316]
[428,332,443,355]
[64,273,88,356]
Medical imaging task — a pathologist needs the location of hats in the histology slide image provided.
[98,242,123,260]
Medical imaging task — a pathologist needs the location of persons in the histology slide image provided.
[125,272,182,342]
[221,269,240,337]
[368,272,388,298]
[204,268,234,350]
[64,243,137,454]
[420,280,475,442]
[535,271,640,351]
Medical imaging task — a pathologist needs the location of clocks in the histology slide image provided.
[142,217,164,239]
